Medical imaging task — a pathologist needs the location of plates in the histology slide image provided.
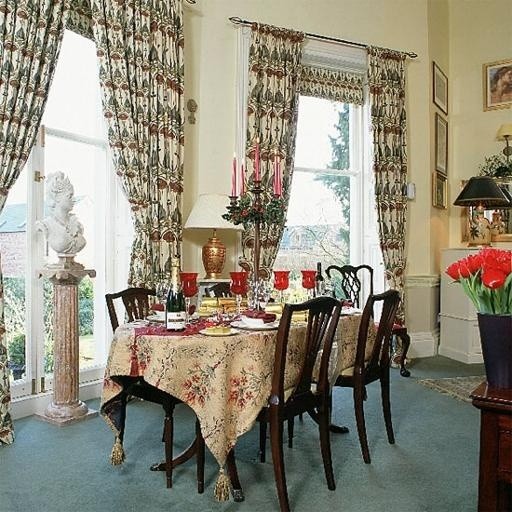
[229,320,280,331]
[198,328,239,338]
[148,315,165,323]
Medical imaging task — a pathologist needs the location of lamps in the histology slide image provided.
[452,175,510,246]
[496,124,512,165]
[183,193,244,280]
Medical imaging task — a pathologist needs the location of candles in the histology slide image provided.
[231,152,236,196]
[255,137,261,180]
[241,159,245,196]
[274,149,281,196]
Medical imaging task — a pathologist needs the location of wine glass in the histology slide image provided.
[273,270,290,308]
[179,272,199,327]
[300,270,318,300]
[230,272,249,318]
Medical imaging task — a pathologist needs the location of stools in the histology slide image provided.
[375,320,411,377]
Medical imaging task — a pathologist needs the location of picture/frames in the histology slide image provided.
[433,61,448,116]
[482,58,512,113]
[432,171,448,209]
[435,112,449,176]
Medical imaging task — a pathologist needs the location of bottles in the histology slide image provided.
[165,254,187,332]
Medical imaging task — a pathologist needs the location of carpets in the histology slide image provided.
[415,375,486,403]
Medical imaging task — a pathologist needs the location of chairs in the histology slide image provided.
[338,288,401,468]
[105,287,193,488]
[204,282,232,298]
[325,262,374,401]
[193,294,344,512]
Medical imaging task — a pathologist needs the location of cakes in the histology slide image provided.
[206,326,230,333]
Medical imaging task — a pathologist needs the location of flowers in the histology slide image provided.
[445,244,512,314]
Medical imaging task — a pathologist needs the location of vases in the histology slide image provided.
[477,311,512,388]
